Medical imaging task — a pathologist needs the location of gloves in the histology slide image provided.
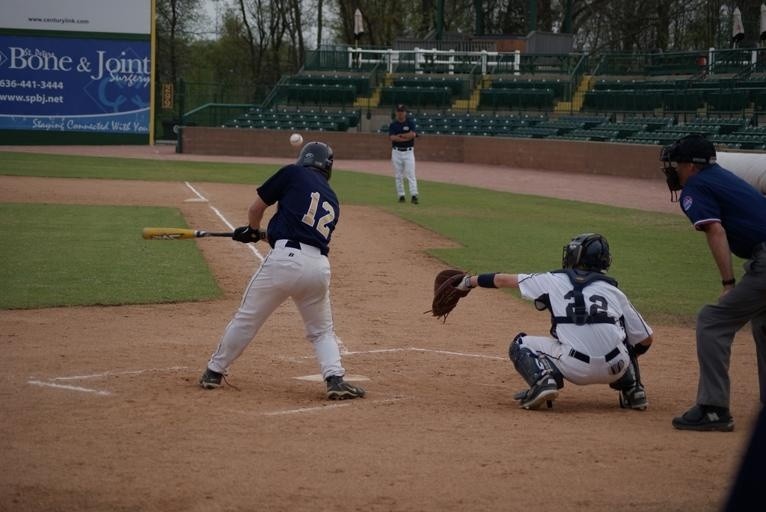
[232,224,266,243]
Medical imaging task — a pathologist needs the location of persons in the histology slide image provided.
[456,233,653,409]
[386,104,419,205]
[660,133,766,431]
[200,141,364,401]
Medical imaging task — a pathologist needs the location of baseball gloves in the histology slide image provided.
[431,270,470,317]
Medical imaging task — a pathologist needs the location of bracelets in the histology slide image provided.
[723,278,736,285]
[398,135,401,138]
[478,272,497,288]
[464,275,474,289]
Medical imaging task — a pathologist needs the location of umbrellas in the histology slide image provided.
[732,6,744,48]
[353,8,364,49]
[759,3,766,36]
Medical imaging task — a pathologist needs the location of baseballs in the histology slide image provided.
[290,133,302,146]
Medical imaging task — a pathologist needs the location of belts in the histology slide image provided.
[394,147,412,151]
[569,340,626,363]
[269,240,328,256]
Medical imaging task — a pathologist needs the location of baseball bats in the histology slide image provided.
[143,227,257,241]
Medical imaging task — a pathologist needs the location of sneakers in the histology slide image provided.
[519,374,558,409]
[400,196,405,203]
[618,386,648,408]
[326,382,364,400]
[411,196,419,205]
[672,405,736,432]
[199,368,223,389]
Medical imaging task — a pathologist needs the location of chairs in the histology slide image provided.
[224,105,376,134]
[477,73,569,112]
[579,76,764,108]
[270,64,383,109]
[376,71,476,105]
[388,106,764,153]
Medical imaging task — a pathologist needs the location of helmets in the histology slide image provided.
[296,141,333,179]
[660,134,717,202]
[562,233,612,274]
